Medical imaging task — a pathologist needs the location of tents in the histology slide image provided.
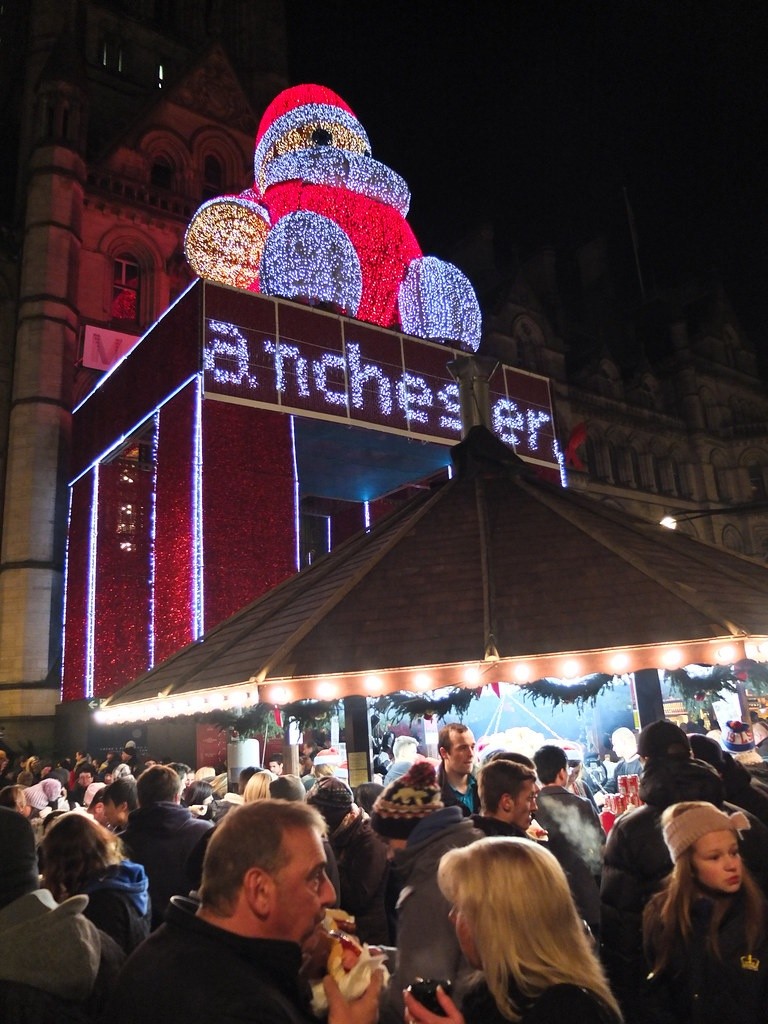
[86,427,768,784]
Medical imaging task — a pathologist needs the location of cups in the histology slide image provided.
[407,979,455,1017]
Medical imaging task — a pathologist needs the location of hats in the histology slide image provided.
[371,761,445,837]
[721,720,755,751]
[124,748,136,755]
[310,776,353,809]
[628,719,690,759]
[23,778,61,810]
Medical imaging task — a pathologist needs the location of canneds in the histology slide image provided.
[605,774,645,815]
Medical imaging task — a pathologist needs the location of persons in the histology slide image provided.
[402,835,623,1024]
[102,802,383,1024]
[0,711,768,1024]
